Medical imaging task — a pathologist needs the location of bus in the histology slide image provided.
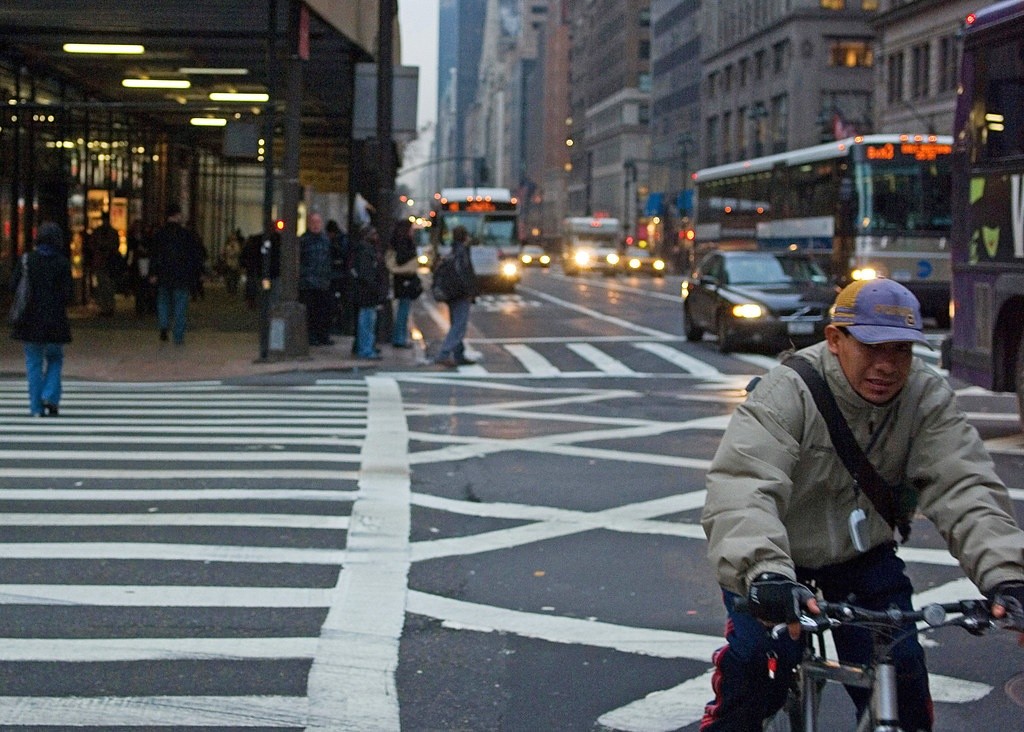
[412,188,550,294]
[689,0,1024,422]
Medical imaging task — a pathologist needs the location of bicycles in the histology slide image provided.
[763,599,1019,732]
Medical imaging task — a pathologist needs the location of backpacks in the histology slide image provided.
[431,254,464,301]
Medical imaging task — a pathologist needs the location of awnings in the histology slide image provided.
[644,191,666,216]
[673,189,694,217]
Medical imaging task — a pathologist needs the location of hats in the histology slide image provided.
[832,275,932,352]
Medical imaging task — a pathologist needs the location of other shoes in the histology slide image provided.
[352,346,381,354]
[393,340,414,348]
[43,401,58,417]
[454,358,476,365]
[437,359,457,371]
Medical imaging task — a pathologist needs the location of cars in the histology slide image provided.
[682,248,841,354]
[561,216,666,278]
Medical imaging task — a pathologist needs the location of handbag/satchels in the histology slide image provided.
[404,273,423,299]
[7,252,33,323]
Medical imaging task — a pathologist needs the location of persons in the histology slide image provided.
[9,204,208,419]
[697,275,1024,732]
[241,213,478,367]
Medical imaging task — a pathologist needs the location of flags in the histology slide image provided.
[833,113,857,140]
